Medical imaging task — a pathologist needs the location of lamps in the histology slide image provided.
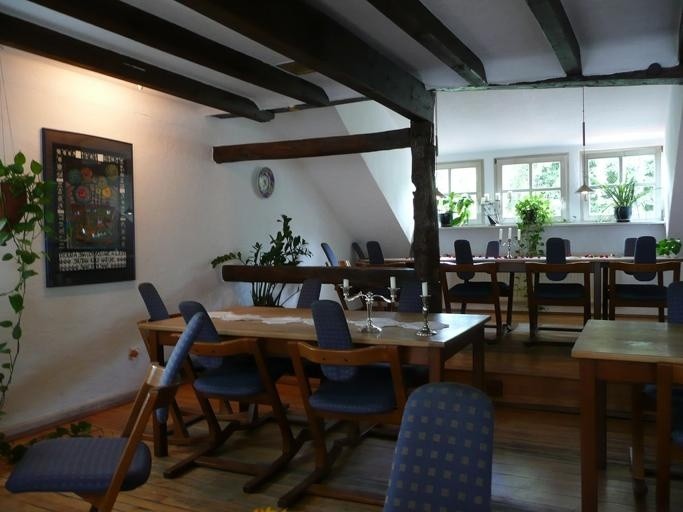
[573,85,595,195]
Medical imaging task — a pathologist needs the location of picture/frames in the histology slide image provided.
[41,126,135,286]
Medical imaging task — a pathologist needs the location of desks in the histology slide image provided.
[570,319,682,510]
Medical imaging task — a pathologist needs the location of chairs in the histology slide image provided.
[320,239,682,342]
[383,383,496,511]
[276,300,417,511]
[163,301,309,491]
[3,313,206,512]
[135,278,494,447]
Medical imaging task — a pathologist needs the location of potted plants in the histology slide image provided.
[600,176,656,223]
[0,141,57,420]
[437,190,473,228]
[511,191,549,313]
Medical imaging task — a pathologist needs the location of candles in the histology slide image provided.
[495,193,499,200]
[507,191,511,198]
[498,228,501,240]
[507,228,511,239]
[481,198,484,204]
[484,193,488,201]
[516,228,520,240]
